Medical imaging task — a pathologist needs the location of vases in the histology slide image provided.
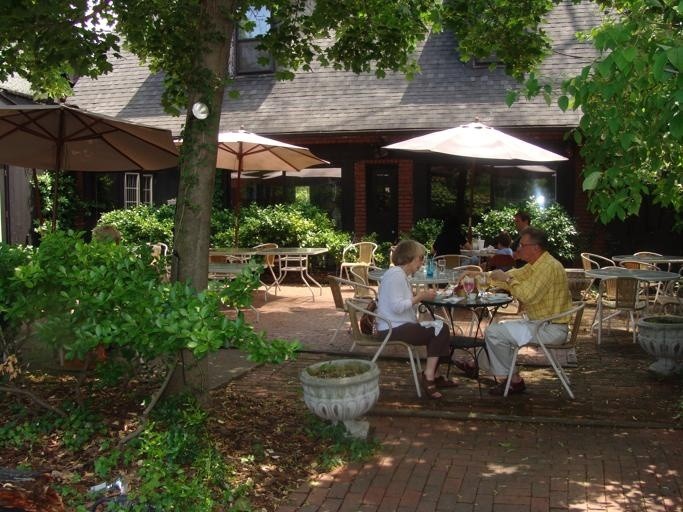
[299,360,380,438]
[637,315,681,378]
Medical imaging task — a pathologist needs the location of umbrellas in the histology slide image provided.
[480,165,557,208]
[0,97,180,233]
[172,124,330,248]
[380,116,569,247]
[262,168,342,203]
[231,171,260,179]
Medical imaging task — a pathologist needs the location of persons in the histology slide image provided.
[460,227,574,397]
[495,231,514,272]
[375,239,458,401]
[431,216,473,265]
[510,210,531,269]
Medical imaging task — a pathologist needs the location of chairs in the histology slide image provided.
[145,242,168,279]
[330,241,682,296]
[344,297,422,401]
[252,243,278,268]
[444,264,485,339]
[503,301,586,399]
[590,275,652,344]
[329,264,386,345]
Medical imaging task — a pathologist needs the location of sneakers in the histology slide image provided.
[489,377,525,395]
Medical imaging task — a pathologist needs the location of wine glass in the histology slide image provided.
[477,272,489,297]
[463,277,474,300]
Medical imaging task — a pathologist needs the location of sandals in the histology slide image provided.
[435,375,458,387]
[418,371,442,400]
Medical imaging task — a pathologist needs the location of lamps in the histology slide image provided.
[190,102,210,120]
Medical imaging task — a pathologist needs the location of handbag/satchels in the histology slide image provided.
[360,301,378,334]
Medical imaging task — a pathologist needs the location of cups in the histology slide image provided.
[438,259,447,274]
[478,239,485,250]
[426,259,435,276]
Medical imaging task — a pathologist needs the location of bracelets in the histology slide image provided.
[506,276,513,283]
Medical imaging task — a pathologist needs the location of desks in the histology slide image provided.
[418,293,513,398]
